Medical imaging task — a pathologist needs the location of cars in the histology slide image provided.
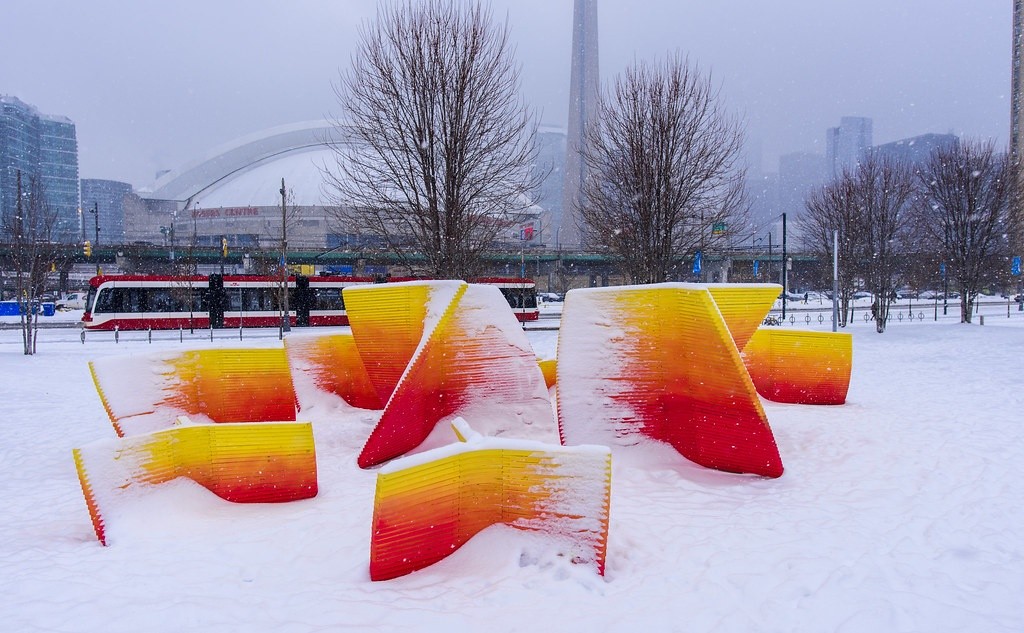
[13,292,87,312]
[780,289,962,301]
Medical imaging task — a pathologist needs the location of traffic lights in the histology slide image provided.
[81,240,92,257]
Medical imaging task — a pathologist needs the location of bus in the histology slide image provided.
[78,273,540,330]
